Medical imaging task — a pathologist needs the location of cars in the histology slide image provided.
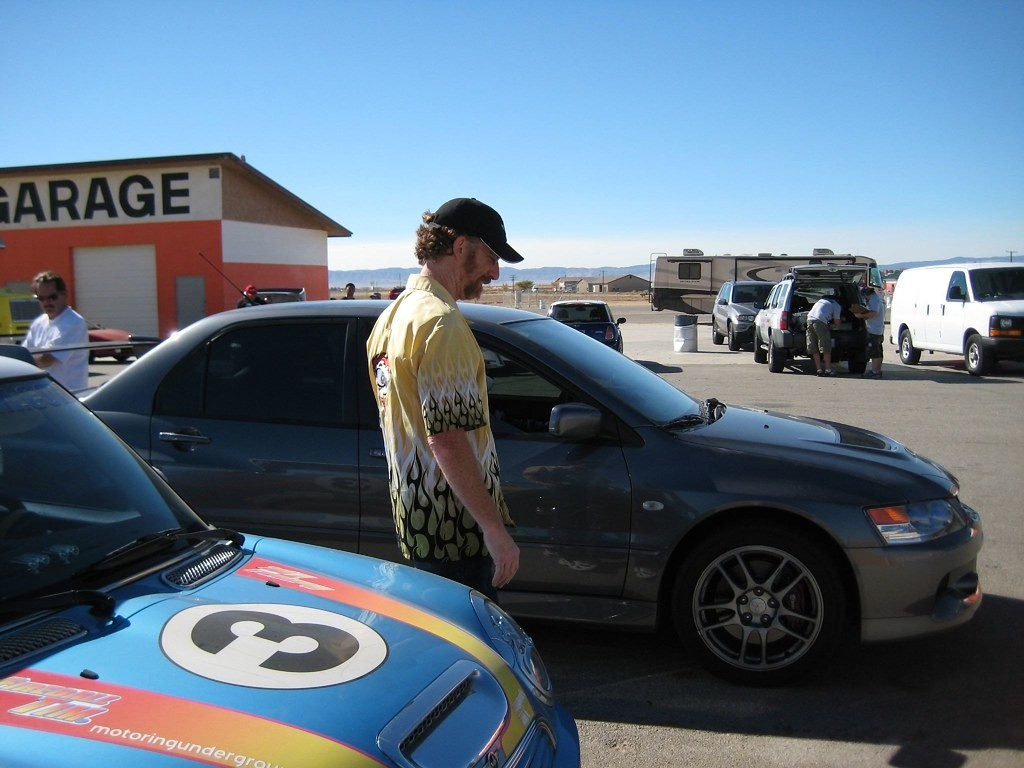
[0,342,583,767]
[83,318,137,364]
[80,297,983,689]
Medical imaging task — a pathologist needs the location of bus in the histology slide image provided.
[649,249,888,314]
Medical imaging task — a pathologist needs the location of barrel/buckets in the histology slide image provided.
[674,314,698,352]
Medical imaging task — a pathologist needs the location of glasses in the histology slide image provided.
[33,293,60,302]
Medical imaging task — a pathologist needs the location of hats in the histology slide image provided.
[425,197,524,264]
[860,286,875,296]
[245,285,257,298]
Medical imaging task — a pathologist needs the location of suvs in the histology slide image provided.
[547,299,627,356]
[753,264,872,373]
[711,280,778,351]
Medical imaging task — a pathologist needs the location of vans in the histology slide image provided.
[890,262,1024,376]
[0,294,45,346]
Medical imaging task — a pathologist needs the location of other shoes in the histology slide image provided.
[815,371,823,377]
[824,370,836,376]
[860,370,882,379]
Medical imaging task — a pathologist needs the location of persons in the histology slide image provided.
[22,271,89,391]
[523,435,666,578]
[367,198,524,606]
[341,282,355,300]
[370,292,382,300]
[235,284,271,309]
[854,287,885,378]
[806,295,844,377]
